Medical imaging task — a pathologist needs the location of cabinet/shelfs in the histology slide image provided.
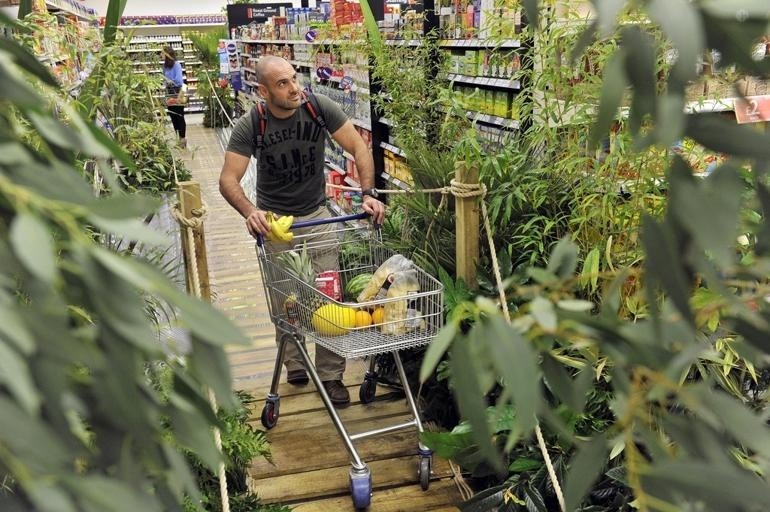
[0,1,113,201]
[96,22,227,114]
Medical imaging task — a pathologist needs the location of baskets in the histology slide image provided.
[165,83,190,106]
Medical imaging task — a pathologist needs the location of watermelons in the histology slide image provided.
[344,273,373,302]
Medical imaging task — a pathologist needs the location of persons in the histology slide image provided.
[155,44,188,150]
[218,56,387,405]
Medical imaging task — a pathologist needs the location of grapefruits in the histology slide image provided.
[312,304,356,336]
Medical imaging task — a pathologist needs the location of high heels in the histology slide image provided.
[174,139,188,149]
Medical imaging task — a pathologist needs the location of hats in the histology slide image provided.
[162,45,177,61]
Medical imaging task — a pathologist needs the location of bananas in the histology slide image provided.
[264,212,294,242]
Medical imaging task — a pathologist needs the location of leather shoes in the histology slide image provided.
[286,369,309,385]
[318,379,351,405]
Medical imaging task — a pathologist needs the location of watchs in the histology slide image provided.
[360,187,380,199]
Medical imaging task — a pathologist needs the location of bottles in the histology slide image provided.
[250,46,258,59]
[113,29,216,114]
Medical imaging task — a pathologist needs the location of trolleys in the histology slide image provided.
[247,209,446,509]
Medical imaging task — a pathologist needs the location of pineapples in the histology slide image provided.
[277,240,327,331]
